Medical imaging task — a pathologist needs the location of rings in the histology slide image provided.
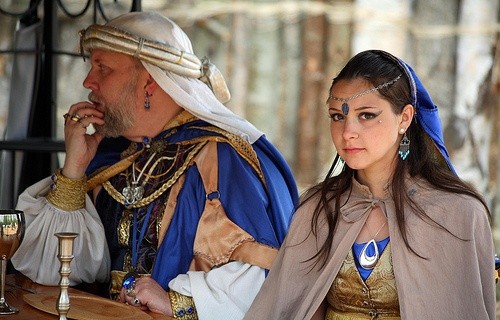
[126,289,136,297]
[122,277,135,288]
[70,114,80,122]
[63,113,71,119]
[133,299,140,304]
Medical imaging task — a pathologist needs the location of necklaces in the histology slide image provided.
[358,219,387,270]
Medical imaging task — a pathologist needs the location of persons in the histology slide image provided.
[9,10,299,320]
[241,50,496,320]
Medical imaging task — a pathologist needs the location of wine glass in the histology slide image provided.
[0,210,26,314]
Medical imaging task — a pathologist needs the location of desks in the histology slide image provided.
[0,274,175,320]
